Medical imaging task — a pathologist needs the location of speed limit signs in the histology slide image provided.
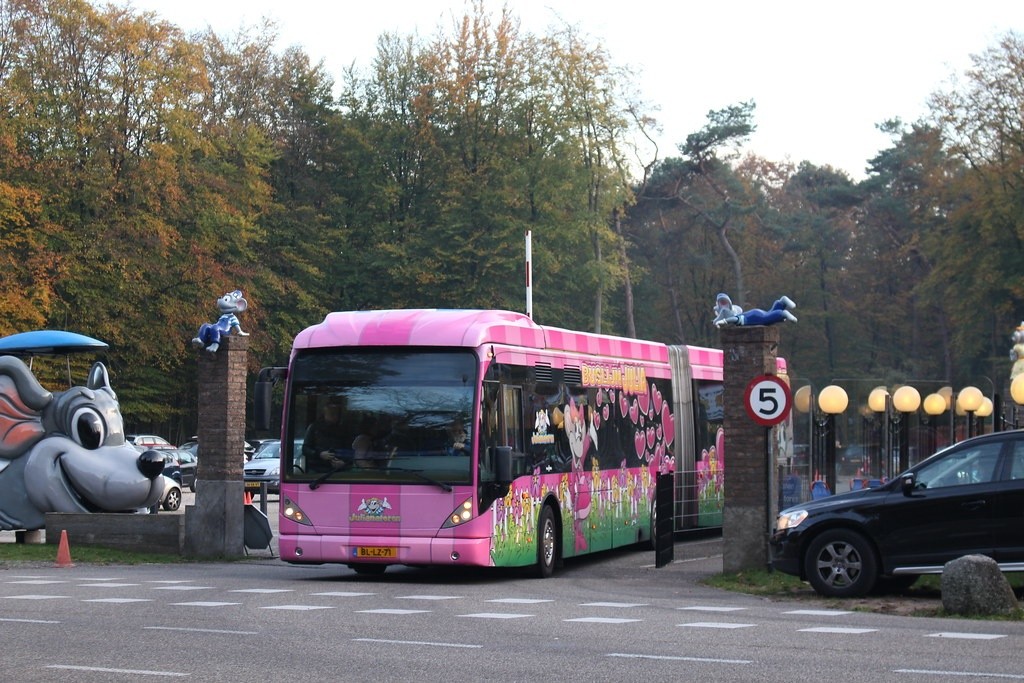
[745,375,793,426]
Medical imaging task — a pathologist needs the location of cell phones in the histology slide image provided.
[329,448,334,453]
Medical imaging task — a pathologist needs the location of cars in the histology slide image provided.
[793,442,919,477]
[772,428,1023,598]
[126,434,305,512]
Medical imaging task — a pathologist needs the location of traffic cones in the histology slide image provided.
[247,491,253,504]
[243,492,248,504]
[50,529,77,568]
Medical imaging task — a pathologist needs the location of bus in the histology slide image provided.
[254,307,794,580]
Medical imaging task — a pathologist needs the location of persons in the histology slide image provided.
[437,420,471,455]
[344,432,379,472]
[303,392,352,473]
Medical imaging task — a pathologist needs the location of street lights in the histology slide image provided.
[973,396,993,435]
[958,387,983,483]
[922,393,946,457]
[893,386,921,473]
[868,388,891,479]
[817,385,848,495]
